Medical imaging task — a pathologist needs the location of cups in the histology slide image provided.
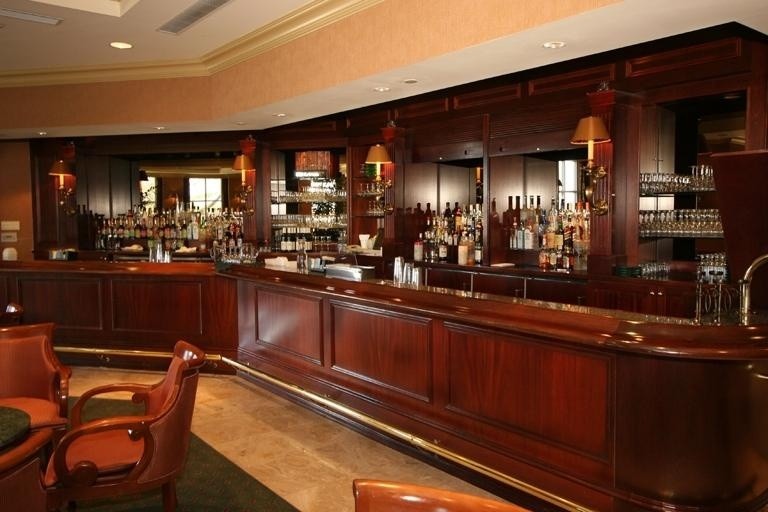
[392,256,423,289]
[271,214,348,228]
[148,242,171,264]
[241,239,259,260]
[358,234,370,248]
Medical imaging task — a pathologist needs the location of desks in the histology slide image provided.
[0,398,78,511]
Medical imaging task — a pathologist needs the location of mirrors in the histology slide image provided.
[439,158,483,215]
[656,106,747,211]
[524,150,590,216]
[271,151,350,196]
[107,154,242,213]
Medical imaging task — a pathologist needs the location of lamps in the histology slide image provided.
[232,155,256,217]
[365,145,393,214]
[570,115,611,216]
[48,160,76,216]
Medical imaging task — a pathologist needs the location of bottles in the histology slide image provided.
[77,199,242,255]
[491,199,500,223]
[508,195,590,270]
[280,226,312,252]
[414,202,483,263]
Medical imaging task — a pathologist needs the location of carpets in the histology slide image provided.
[41,396,302,511]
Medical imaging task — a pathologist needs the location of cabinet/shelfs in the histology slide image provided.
[639,188,725,240]
[271,199,347,230]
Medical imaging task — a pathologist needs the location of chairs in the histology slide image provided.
[0,323,73,446]
[37,340,207,510]
[0,301,24,325]
[351,479,527,512]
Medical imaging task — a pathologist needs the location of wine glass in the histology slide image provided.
[640,208,723,234]
[697,253,726,282]
[638,262,672,281]
[640,164,715,193]
[358,182,385,214]
[271,189,347,202]
[313,228,348,255]
[297,238,308,275]
